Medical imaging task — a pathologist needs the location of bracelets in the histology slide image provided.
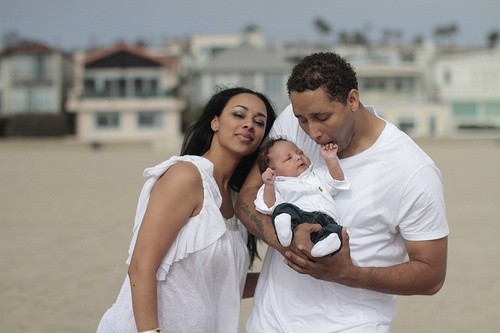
[138,327,162,333]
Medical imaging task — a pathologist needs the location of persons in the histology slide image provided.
[253,135,353,258]
[232,52,450,332]
[95,86,276,333]
